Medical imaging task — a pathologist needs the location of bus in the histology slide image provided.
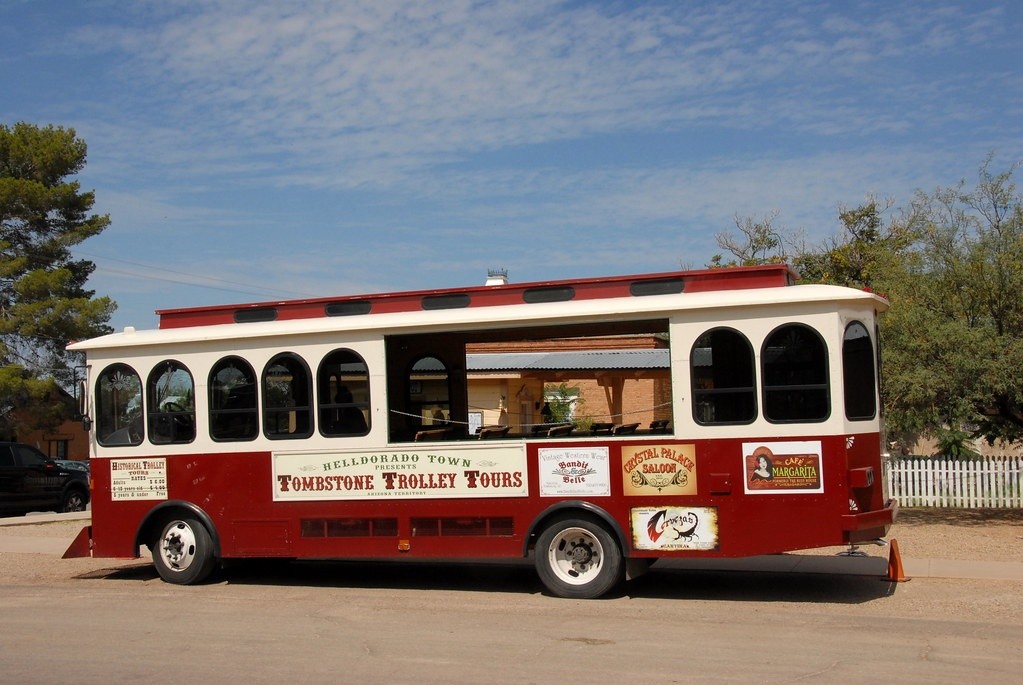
[57,265,895,600]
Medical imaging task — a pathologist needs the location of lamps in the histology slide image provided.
[536,397,551,416]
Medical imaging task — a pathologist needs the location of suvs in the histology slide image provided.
[0,444,91,518]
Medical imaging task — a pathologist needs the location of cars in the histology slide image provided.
[55,459,89,473]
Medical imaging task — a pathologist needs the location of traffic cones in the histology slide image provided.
[880,537,913,582]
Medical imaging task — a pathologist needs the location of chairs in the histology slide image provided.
[413,421,673,442]
